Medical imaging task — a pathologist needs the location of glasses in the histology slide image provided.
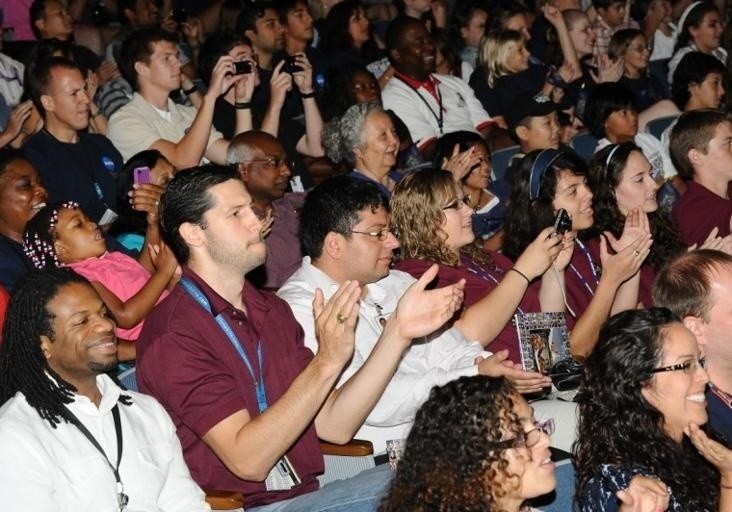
[241,156,294,170]
[444,192,472,212]
[628,41,653,55]
[346,224,393,241]
[647,350,708,378]
[489,419,556,449]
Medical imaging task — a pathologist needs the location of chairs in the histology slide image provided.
[115,52,731,512]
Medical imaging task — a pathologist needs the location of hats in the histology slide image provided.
[508,91,572,124]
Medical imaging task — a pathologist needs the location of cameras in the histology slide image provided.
[230,61,251,75]
[274,55,304,72]
[552,208,572,233]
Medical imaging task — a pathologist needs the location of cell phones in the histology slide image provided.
[134,167,150,185]
